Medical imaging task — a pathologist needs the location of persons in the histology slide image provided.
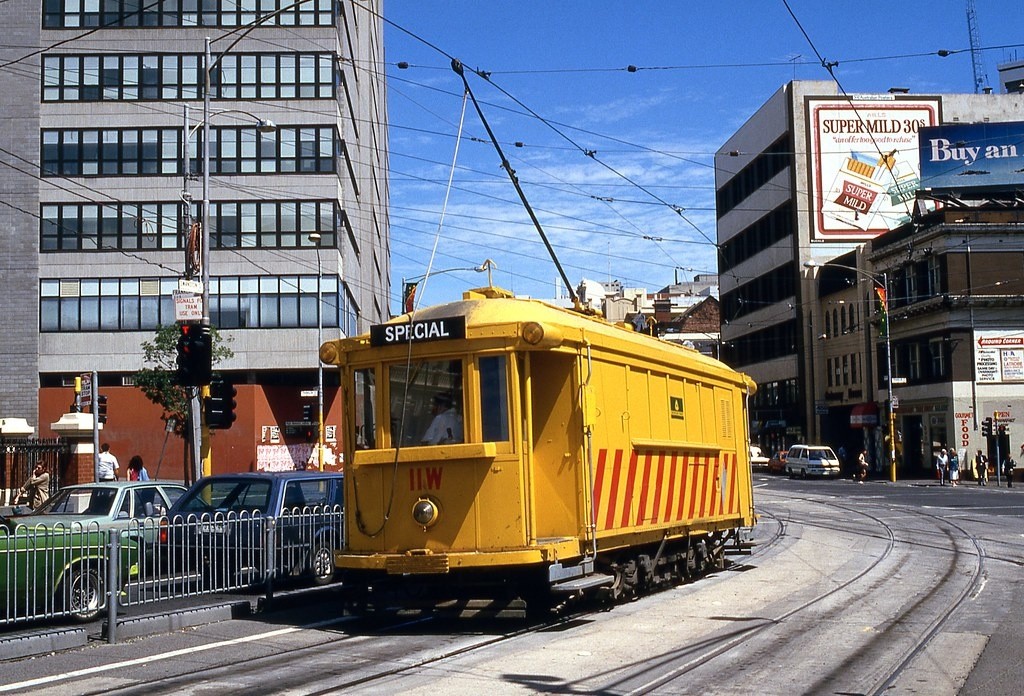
[126,455,149,482]
[936,448,1017,487]
[840,446,870,484]
[99,443,120,482]
[13,460,52,514]
[420,392,465,445]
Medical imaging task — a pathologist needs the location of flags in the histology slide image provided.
[877,288,886,311]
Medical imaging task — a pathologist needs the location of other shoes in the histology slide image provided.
[857,481,863,485]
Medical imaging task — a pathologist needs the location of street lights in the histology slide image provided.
[401,266,486,317]
[307,232,324,472]
[181,100,276,286]
[804,262,896,483]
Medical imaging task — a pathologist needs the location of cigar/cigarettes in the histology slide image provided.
[847,154,896,185]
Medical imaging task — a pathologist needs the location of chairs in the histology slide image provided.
[284,487,304,509]
[107,494,131,514]
[86,494,112,515]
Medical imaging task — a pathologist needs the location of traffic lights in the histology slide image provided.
[98,395,108,424]
[175,324,211,386]
[982,417,992,437]
[208,381,237,429]
[999,425,1006,440]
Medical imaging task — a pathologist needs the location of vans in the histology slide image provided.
[784,444,840,479]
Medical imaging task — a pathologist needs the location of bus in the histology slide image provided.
[317,287,757,622]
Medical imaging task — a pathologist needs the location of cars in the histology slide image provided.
[10,481,211,553]
[0,514,139,623]
[768,451,789,474]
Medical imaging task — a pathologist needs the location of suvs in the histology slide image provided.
[158,472,346,586]
